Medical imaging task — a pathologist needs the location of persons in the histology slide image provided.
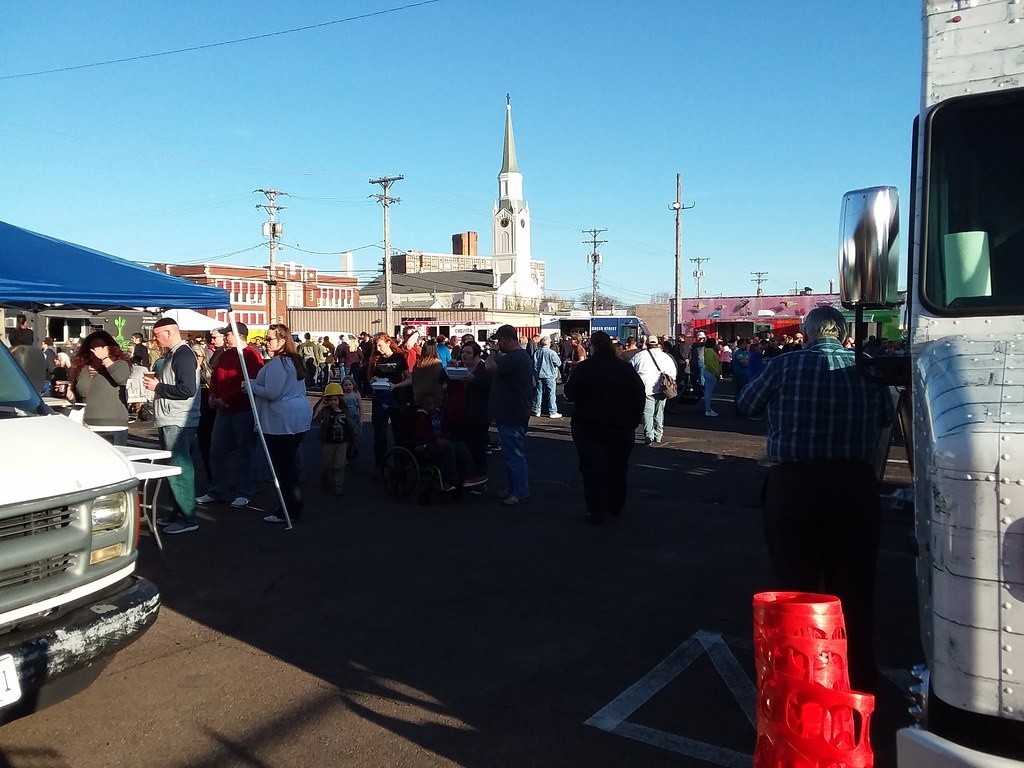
[42,322,911,534]
[8,314,34,345]
[736,306,895,698]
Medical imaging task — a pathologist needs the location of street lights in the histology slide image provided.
[667,201,696,350]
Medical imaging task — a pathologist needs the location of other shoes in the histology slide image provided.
[705,410,718,416]
[644,441,652,445]
[496,489,513,498]
[463,476,488,487]
[195,494,217,504]
[654,437,661,442]
[443,484,456,491]
[231,497,253,508]
[584,511,603,526]
[550,413,562,418]
[264,516,295,522]
[503,495,530,505]
[535,413,540,418]
[611,508,622,518]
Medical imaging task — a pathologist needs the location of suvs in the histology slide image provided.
[0,341,162,727]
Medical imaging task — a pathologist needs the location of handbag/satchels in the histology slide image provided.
[661,374,677,399]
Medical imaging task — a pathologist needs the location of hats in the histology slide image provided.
[697,332,706,341]
[491,325,517,339]
[647,335,658,344]
[217,322,248,335]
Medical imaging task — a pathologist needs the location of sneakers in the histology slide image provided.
[157,514,184,525]
[163,519,198,533]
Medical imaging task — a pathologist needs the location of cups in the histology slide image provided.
[56,381,69,397]
[144,372,155,389]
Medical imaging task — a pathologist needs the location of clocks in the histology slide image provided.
[521,219,526,228]
[500,218,509,228]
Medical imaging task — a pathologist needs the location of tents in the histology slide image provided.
[160,308,228,331]
[0,221,293,530]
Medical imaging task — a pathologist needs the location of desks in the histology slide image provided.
[112,444,182,550]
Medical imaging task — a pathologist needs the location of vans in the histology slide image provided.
[292,331,361,353]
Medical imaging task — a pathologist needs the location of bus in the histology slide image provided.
[838,1,1023,766]
[399,315,662,384]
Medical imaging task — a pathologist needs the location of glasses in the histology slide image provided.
[153,330,168,337]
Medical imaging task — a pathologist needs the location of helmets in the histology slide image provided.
[322,383,345,396]
